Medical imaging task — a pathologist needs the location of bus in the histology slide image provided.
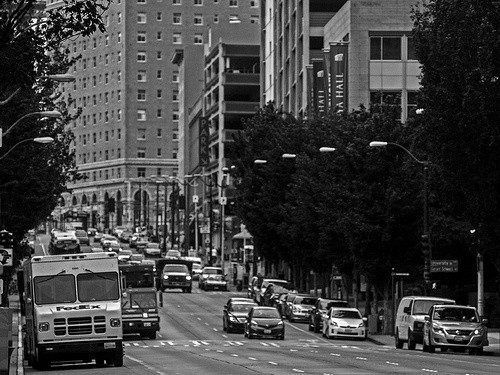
[118,258,165,342]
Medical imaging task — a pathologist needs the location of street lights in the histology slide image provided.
[369,140,430,298]
[117,164,237,276]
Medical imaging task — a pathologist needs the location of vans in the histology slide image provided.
[392,296,458,351]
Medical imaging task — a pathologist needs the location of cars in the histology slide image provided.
[308,297,351,333]
[322,306,369,340]
[35,219,230,293]
[243,305,287,340]
[221,274,319,333]
[422,303,489,355]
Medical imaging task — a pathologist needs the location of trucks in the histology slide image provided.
[17,250,125,371]
